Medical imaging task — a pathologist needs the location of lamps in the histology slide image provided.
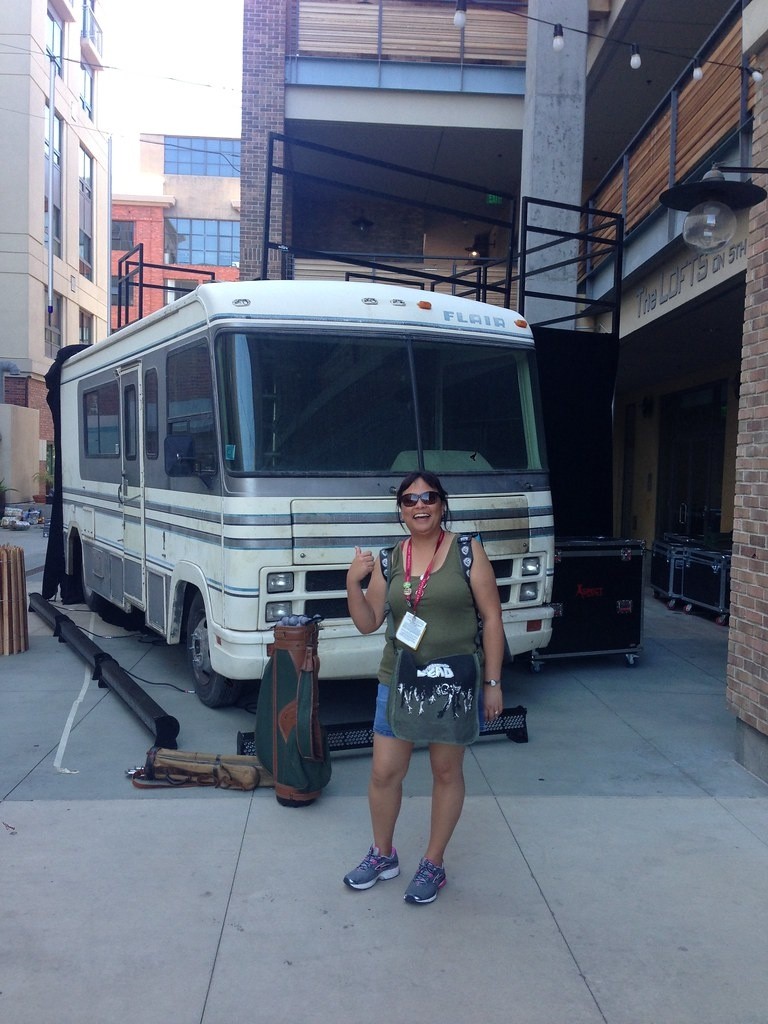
[465,241,495,261]
[659,161,768,254]
[350,209,374,232]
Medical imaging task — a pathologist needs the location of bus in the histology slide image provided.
[59,280,555,711]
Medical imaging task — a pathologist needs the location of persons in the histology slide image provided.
[343,470,505,904]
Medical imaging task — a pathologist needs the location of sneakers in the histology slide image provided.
[403,856,448,903]
[343,842,400,890]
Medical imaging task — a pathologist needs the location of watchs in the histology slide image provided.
[484,679,502,687]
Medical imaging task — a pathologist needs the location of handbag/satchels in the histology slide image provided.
[386,645,480,745]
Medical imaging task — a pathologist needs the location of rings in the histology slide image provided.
[494,711,498,714]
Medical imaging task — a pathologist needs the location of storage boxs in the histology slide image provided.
[650,540,732,625]
[531,537,646,672]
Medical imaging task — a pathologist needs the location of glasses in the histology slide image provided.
[397,490,442,507]
